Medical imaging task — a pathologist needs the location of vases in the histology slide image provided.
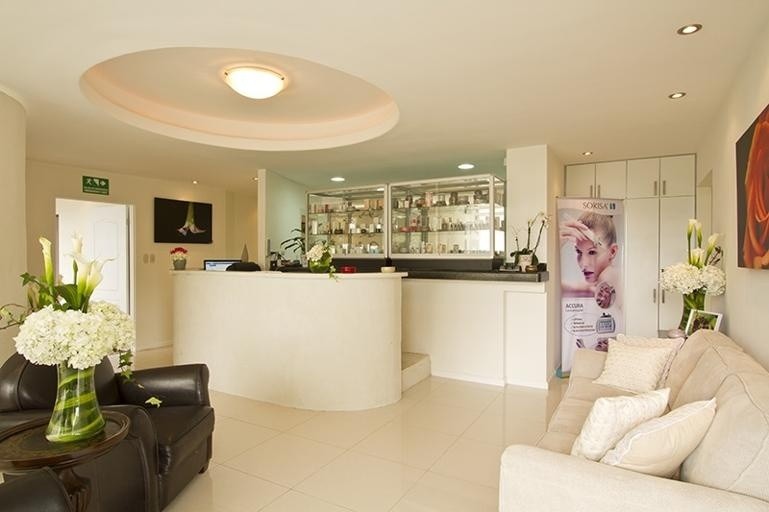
[309,258,330,273]
[518,252,534,272]
[173,261,185,270]
[43,360,106,443]
[381,266,397,273]
[675,288,707,331]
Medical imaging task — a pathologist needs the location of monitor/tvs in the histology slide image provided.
[204,259,242,271]
[153,196,212,243]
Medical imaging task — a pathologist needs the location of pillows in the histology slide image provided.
[592,336,673,393]
[595,395,718,477]
[614,332,685,391]
[570,387,670,462]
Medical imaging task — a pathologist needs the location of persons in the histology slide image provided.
[558,211,622,297]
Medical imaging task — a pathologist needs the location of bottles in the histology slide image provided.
[441,217,463,231]
[405,190,413,207]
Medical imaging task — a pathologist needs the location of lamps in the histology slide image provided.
[217,63,291,101]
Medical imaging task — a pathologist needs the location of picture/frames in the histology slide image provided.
[683,307,724,337]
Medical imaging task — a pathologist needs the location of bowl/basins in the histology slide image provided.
[381,267,396,273]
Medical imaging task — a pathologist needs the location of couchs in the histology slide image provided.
[498,329,768,511]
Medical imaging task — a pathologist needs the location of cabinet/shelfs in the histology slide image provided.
[564,159,627,197]
[625,197,696,337]
[305,184,389,260]
[389,173,505,260]
[628,154,696,196]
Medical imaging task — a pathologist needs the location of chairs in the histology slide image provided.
[0,351,216,512]
[0,465,75,511]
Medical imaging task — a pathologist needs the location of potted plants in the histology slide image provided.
[280,223,319,268]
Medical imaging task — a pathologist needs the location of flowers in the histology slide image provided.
[657,215,728,333]
[305,240,340,283]
[0,231,164,443]
[170,247,192,261]
[510,209,553,268]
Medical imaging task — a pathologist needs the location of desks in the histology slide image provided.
[657,329,670,338]
[0,409,157,511]
